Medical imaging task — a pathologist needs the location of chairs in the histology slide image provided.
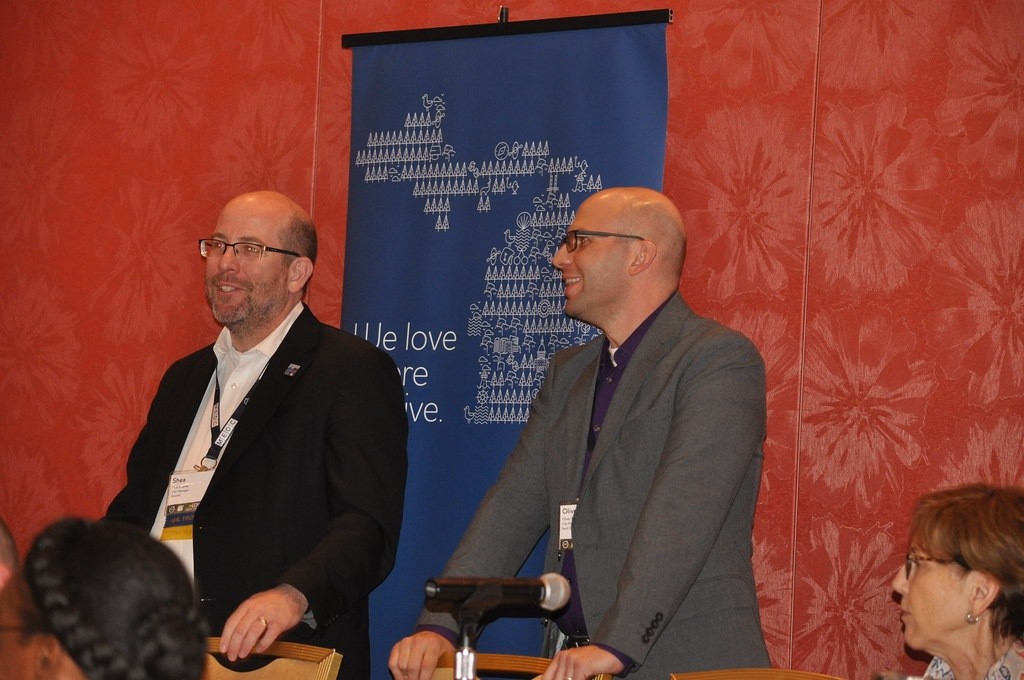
[431,651,612,680]
[203,638,343,680]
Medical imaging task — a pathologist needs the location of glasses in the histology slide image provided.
[559,230,646,253]
[906,552,956,580]
[198,239,300,263]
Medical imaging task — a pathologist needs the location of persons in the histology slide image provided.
[892,486,1024,680]
[389,188,772,680]
[0,190,407,680]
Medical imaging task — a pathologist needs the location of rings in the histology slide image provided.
[257,615,268,627]
[562,676,573,680]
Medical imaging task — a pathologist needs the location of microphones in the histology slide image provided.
[424,571,571,609]
